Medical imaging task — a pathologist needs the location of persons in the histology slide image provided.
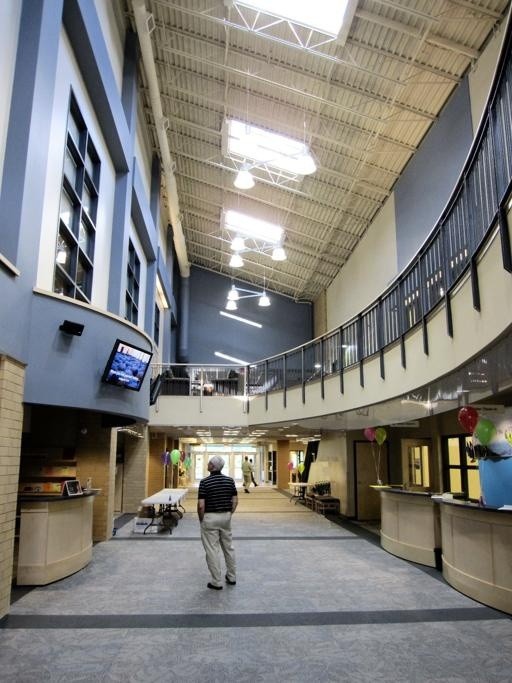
[241,456,255,493]
[174,355,214,395]
[248,459,258,486]
[198,455,238,591]
[472,444,494,507]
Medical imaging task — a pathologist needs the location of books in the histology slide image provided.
[374,427,387,446]
[363,427,376,444]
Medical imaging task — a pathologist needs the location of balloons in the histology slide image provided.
[184,456,190,471]
[297,461,306,475]
[180,450,185,463]
[170,449,180,465]
[456,405,477,437]
[286,462,294,471]
[161,452,170,464]
[475,417,497,450]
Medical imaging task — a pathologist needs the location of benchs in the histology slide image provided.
[303,492,340,516]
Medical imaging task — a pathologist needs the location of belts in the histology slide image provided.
[204,510,231,513]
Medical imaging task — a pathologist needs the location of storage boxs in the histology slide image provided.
[133,512,165,533]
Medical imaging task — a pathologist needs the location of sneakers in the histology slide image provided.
[243,483,259,493]
[207,583,223,590]
[225,575,236,585]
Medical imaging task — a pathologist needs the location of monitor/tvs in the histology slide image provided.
[101,338,153,391]
[151,374,163,404]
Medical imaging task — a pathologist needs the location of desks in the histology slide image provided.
[287,482,312,505]
[139,487,190,535]
[213,377,238,396]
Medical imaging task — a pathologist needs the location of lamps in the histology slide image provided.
[224,72,317,311]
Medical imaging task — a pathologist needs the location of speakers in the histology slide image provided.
[62,320,84,336]
